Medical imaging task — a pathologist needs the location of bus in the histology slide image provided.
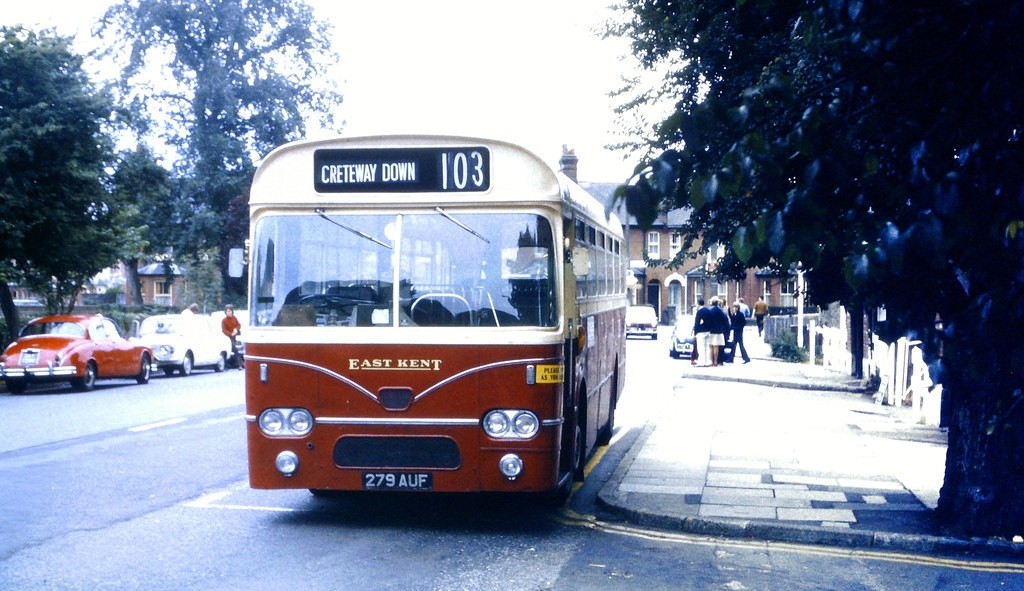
[227,132,631,507]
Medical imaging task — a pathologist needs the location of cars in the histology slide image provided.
[626,303,660,341]
[0,312,155,396]
[669,313,698,359]
[128,308,271,378]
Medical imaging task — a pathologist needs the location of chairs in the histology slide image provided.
[412,281,477,325]
[325,287,376,314]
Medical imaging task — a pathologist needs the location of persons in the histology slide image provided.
[181,303,200,316]
[694,297,713,367]
[222,304,244,370]
[691,325,697,364]
[719,298,731,327]
[626,287,635,306]
[725,302,750,364]
[733,298,750,318]
[751,295,769,336]
[709,296,730,366]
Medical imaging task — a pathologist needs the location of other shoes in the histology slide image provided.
[742,359,751,364]
[725,358,733,362]
[691,362,697,365]
